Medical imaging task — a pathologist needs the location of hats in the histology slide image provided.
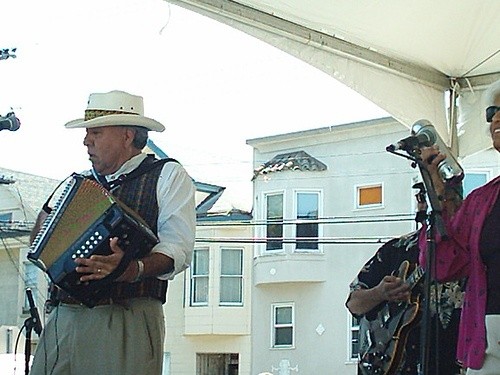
[64,90,166,133]
[412,180,463,198]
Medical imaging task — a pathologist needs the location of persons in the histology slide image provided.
[28,91,197,375]
[344,182,465,375]
[418,80,500,375]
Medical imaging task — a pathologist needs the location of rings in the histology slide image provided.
[97,268,101,273]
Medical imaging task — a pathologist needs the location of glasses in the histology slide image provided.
[486,106,500,122]
[414,193,445,203]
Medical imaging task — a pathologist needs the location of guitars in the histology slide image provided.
[357,263,427,375]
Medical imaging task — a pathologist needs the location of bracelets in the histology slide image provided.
[130,259,144,283]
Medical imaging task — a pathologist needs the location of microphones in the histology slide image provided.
[386,128,438,152]
[0,116,21,131]
